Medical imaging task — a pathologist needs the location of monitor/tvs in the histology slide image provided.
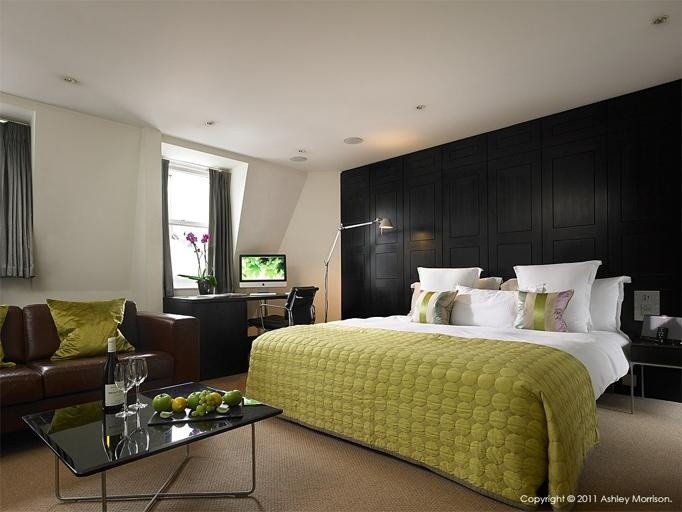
[238,254,287,296]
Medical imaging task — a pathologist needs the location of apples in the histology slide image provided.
[152,393,172,413]
[188,422,199,429]
[153,423,172,433]
[187,392,201,410]
[223,390,242,407]
[225,419,242,425]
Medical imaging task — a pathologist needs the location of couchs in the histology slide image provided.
[0,287,201,443]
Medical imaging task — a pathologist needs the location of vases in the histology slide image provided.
[198,282,214,296]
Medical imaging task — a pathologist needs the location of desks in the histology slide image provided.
[168,290,289,380]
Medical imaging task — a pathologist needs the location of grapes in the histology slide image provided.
[199,422,216,432]
[191,390,215,416]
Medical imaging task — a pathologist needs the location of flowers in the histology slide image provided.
[173,227,216,284]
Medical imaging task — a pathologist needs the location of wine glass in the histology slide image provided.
[115,417,139,460]
[115,362,138,418]
[128,357,149,408]
[128,408,149,455]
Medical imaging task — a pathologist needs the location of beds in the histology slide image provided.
[246,308,638,512]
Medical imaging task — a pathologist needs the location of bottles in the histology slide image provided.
[102,336,125,414]
[101,410,127,461]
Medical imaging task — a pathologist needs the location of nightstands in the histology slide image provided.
[624,340,682,414]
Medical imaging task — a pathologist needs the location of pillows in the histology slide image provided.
[0,303,15,366]
[513,257,602,333]
[39,296,136,359]
[410,288,457,325]
[416,264,484,289]
[451,284,518,329]
[408,277,501,291]
[503,276,633,333]
[511,288,574,331]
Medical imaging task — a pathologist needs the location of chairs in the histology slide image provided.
[248,285,319,334]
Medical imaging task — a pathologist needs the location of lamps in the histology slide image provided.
[321,217,393,323]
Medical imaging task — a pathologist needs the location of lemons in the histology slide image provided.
[216,421,225,427]
[171,396,186,412]
[174,422,185,427]
[210,392,222,406]
